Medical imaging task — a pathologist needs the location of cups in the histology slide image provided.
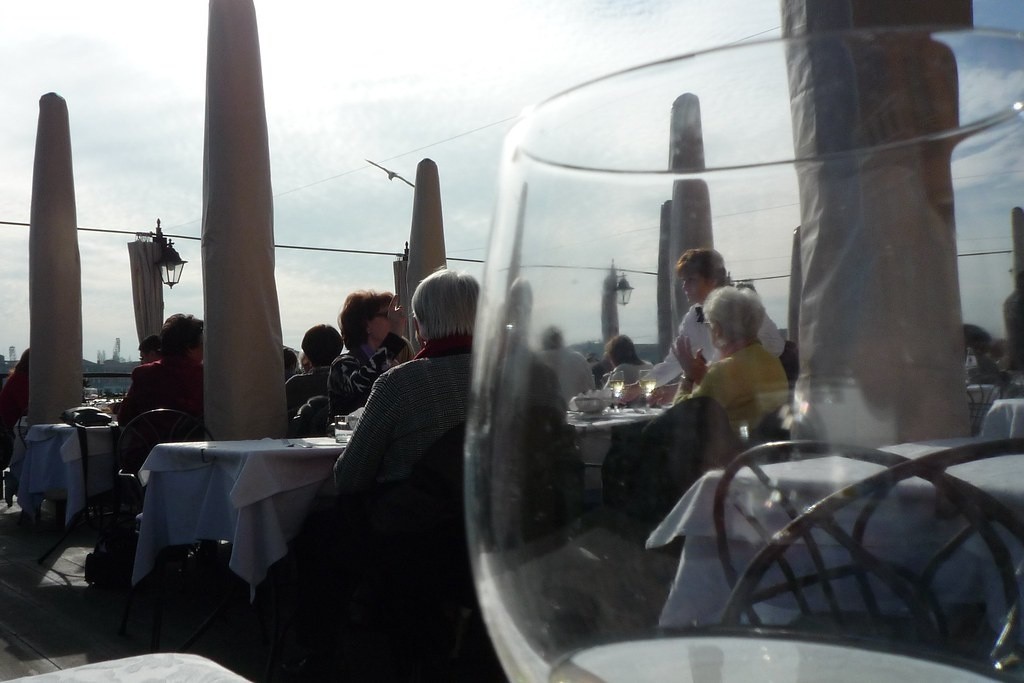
[335,416,358,442]
[467,26,1024,682]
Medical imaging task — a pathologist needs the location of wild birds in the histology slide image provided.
[369,161,416,191]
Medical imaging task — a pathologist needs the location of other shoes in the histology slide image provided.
[281,654,350,682]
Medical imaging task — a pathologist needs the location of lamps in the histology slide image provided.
[614,274,635,306]
[154,241,187,289]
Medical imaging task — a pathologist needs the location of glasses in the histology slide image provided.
[376,311,388,318]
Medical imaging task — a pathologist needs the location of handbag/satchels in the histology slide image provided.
[86,529,140,590]
[59,406,112,426]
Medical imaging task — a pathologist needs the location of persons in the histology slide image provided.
[282,262,583,679]
[117,317,218,576]
[0,348,29,507]
[962,323,1012,436]
[109,333,162,415]
[284,246,799,534]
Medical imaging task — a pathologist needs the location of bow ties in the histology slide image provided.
[695,307,705,324]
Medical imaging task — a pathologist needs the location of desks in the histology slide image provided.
[131,437,353,668]
[646,437,1024,647]
[979,398,1024,439]
[967,384,999,430]
[19,423,126,527]
[560,406,665,525]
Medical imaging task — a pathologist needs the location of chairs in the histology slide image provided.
[712,442,1016,653]
[720,441,1024,672]
[966,382,1003,434]
[117,408,215,559]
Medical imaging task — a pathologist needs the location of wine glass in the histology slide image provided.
[639,370,656,414]
[609,370,625,415]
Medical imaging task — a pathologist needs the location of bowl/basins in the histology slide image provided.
[574,398,605,414]
